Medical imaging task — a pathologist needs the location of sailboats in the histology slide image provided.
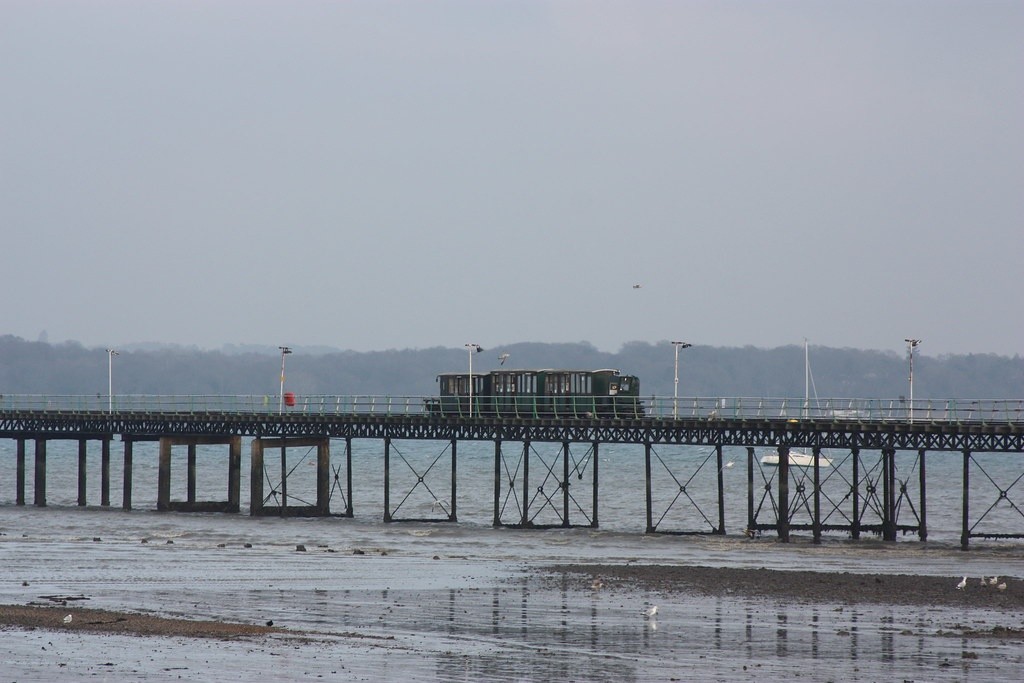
[760,337,835,467]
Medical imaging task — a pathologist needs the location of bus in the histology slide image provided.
[424,369,646,418]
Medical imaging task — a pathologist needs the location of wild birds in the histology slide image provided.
[955,573,1007,593]
[62,613,73,625]
[639,605,660,620]
[631,282,644,291]
[715,459,744,474]
[497,353,511,366]
[265,619,274,628]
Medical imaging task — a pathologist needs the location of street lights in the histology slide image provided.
[465,344,484,417]
[106,349,120,414]
[905,339,921,423]
[279,347,292,415]
[672,341,692,420]
